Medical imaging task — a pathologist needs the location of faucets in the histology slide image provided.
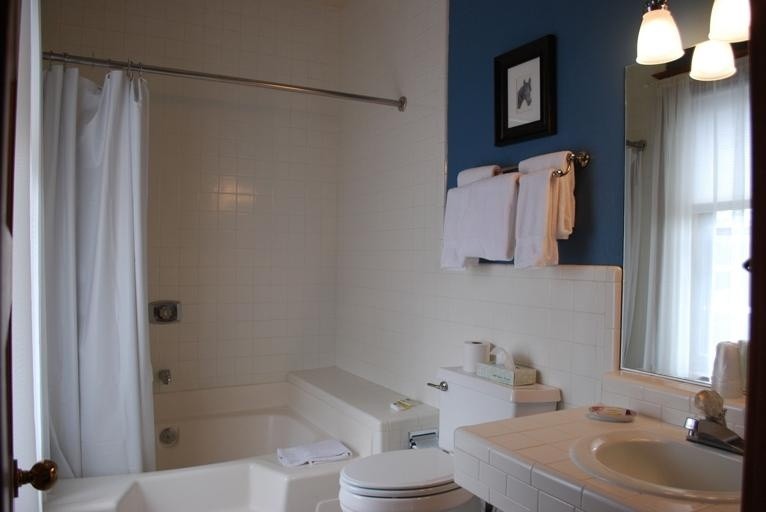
[683,417,744,454]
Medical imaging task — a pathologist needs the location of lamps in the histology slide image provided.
[635,0,686,66]
[707,0,751,44]
[689,40,737,82]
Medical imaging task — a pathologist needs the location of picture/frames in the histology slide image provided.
[493,33,557,147]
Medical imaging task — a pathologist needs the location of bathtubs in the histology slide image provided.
[43,367,439,512]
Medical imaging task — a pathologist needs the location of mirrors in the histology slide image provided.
[618,42,750,396]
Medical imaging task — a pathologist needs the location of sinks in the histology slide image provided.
[570,429,743,503]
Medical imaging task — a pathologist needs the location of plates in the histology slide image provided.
[588,404,637,421]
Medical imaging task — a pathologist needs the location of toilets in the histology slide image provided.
[337,366,561,512]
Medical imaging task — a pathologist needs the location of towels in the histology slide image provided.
[277,438,352,467]
[457,166,500,188]
[515,169,558,272]
[519,151,575,239]
[440,187,480,271]
